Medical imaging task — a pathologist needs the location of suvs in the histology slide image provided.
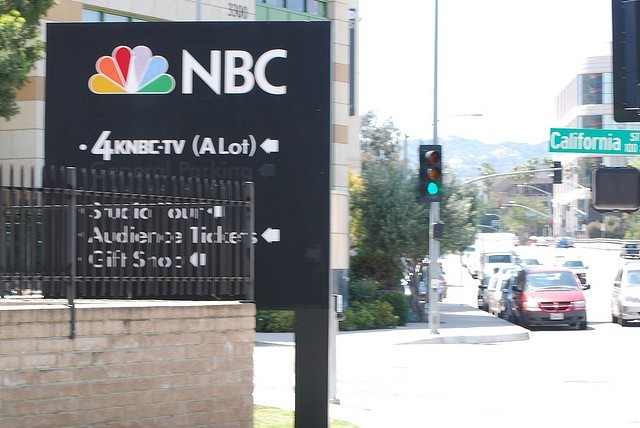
[554,237,574,247]
[611,262,640,325]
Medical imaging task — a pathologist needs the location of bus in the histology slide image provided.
[483,253,513,273]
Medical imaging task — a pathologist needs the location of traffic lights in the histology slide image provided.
[554,162,562,184]
[611,1,640,123]
[420,144,442,201]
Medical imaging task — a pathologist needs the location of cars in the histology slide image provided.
[523,258,543,265]
[484,273,499,313]
[535,237,549,246]
[494,276,510,316]
[501,277,517,320]
[561,260,589,285]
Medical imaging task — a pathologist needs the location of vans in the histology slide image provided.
[514,267,587,330]
[478,276,490,309]
[620,243,640,259]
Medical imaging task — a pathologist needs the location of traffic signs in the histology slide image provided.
[549,128,640,157]
